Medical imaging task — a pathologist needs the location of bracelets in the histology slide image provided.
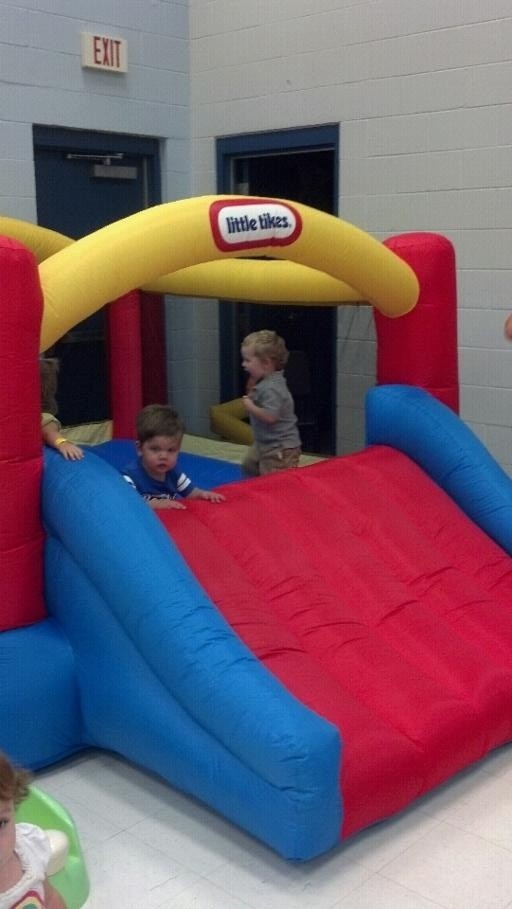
[54,437,74,448]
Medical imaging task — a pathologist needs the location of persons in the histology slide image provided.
[0,752,67,909]
[40,357,86,461]
[118,403,226,510]
[239,329,303,477]
